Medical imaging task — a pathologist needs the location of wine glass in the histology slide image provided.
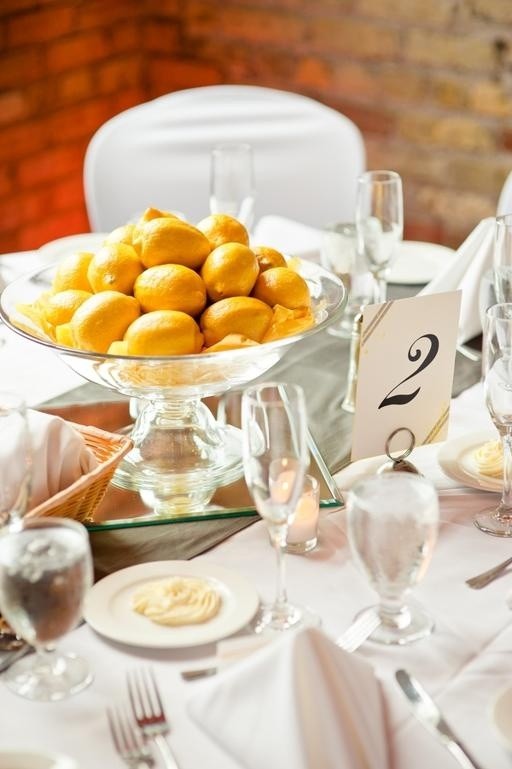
[343,472,441,647]
[0,513,98,702]
[472,305,512,539]
[207,143,255,241]
[355,169,405,303]
[493,212,512,318]
[239,380,324,636]
[478,271,495,329]
[322,220,363,338]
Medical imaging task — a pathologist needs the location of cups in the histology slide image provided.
[265,460,322,553]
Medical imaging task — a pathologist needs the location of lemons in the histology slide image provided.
[46,206,308,355]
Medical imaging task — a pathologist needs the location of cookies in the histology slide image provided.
[476,440,507,478]
[132,578,220,624]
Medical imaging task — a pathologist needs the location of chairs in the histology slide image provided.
[82,79,368,236]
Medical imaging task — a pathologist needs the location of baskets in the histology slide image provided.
[1,408,135,536]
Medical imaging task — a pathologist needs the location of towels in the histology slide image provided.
[411,213,511,342]
[195,629,403,768]
[2,408,104,523]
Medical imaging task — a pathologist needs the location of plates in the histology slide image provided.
[83,557,261,652]
[436,427,506,493]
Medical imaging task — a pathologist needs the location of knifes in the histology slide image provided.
[393,665,486,769]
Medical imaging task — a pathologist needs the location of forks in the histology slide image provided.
[124,667,189,768]
[107,705,154,768]
